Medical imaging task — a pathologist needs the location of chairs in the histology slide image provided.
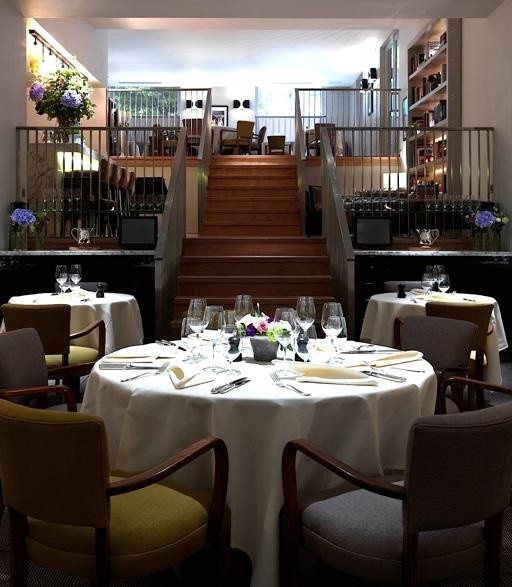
[0,328,73,403]
[150,118,336,156]
[280,376,511,585]
[385,316,485,415]
[1,304,105,385]
[425,301,495,406]
[1,398,252,585]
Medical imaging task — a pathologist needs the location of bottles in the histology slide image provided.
[95,283,105,298]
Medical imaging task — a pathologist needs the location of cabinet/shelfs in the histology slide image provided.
[405,15,462,202]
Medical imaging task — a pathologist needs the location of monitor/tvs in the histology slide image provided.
[119,216,158,250]
[352,216,393,250]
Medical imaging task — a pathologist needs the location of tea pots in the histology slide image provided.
[70,226,95,248]
[416,228,440,248]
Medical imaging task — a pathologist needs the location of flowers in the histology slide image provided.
[473,208,508,229]
[8,207,37,227]
[28,207,55,232]
[26,59,104,133]
[232,311,292,340]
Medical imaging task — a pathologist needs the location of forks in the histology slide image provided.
[269,371,310,397]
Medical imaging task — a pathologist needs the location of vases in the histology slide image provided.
[33,233,47,250]
[66,134,85,144]
[13,229,29,251]
[251,337,281,362]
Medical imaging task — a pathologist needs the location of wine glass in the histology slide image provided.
[188,299,209,349]
[281,311,301,363]
[235,295,255,323]
[202,305,225,360]
[320,303,344,352]
[296,296,314,347]
[218,310,235,356]
[274,308,296,368]
[55,265,81,293]
[420,265,450,299]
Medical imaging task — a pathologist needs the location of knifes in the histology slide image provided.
[220,378,251,395]
[99,364,160,369]
[211,376,247,394]
[360,370,406,383]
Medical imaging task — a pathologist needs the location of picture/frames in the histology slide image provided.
[209,105,228,127]
[367,84,373,115]
